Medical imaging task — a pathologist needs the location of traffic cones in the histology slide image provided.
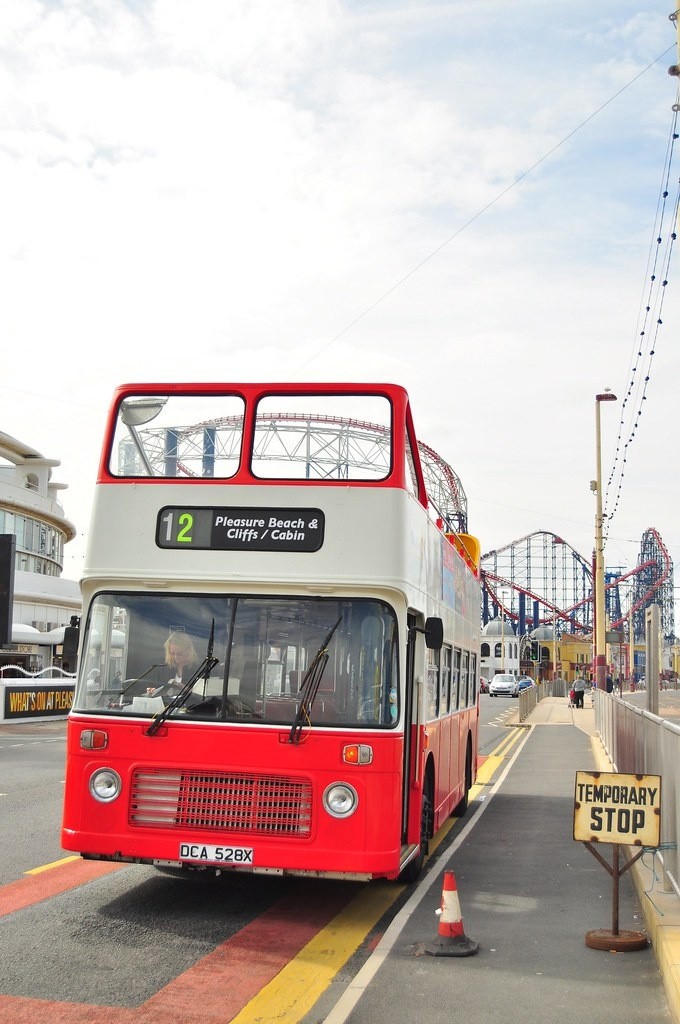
[424,865,478,958]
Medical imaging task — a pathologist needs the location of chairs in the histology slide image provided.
[256,691,338,724]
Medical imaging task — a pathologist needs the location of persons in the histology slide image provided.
[146,631,207,697]
[572,675,586,710]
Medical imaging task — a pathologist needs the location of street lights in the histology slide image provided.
[592,392,618,694]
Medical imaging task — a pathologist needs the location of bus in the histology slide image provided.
[60,377,488,890]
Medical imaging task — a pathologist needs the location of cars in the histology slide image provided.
[486,672,517,695]
